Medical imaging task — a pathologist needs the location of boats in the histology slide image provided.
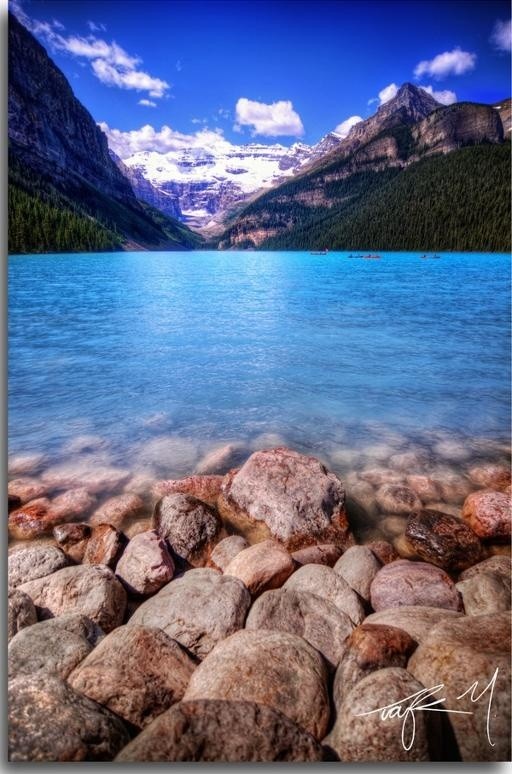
[311,251,327,255]
[349,254,380,259]
[420,255,441,258]
